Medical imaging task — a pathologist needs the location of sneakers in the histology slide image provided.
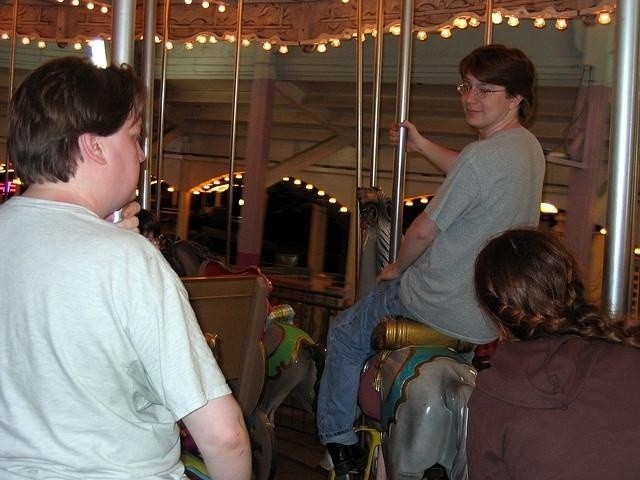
[326,443,368,476]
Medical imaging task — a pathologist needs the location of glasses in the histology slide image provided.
[455,79,511,98]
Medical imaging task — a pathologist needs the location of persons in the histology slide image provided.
[467,226,640,480]
[316,40,553,479]
[1,57,258,480]
